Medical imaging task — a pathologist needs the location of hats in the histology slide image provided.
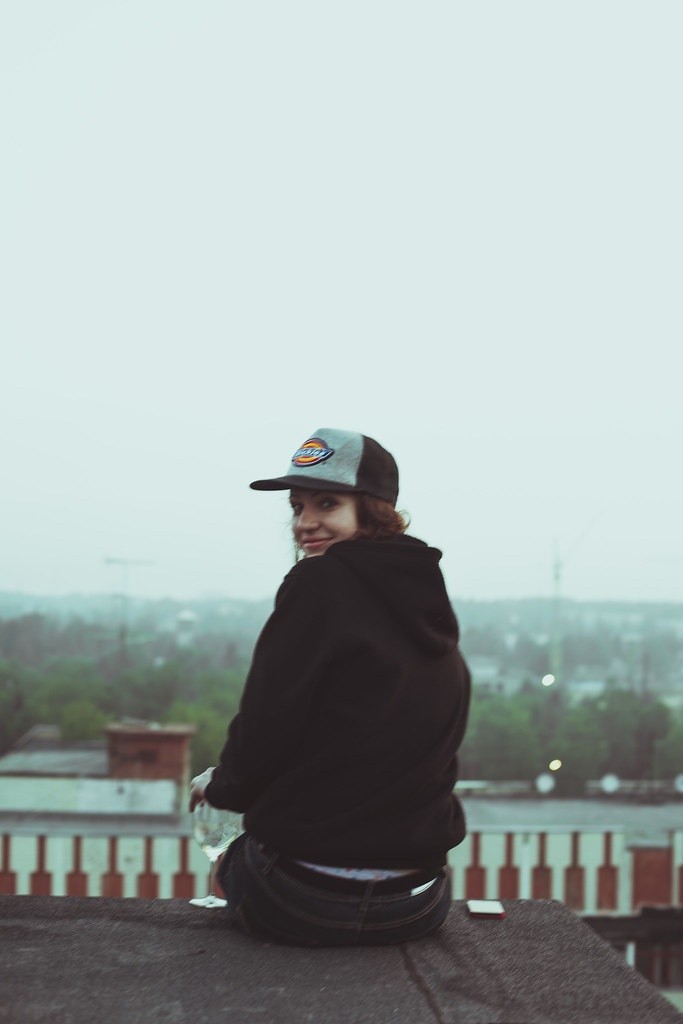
[249,428,399,507]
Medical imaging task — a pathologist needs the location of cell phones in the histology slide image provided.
[466,899,507,920]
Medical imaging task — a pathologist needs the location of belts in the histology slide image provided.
[256,842,443,896]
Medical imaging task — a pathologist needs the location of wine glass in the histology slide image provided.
[189,801,243,909]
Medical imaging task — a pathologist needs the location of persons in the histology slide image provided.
[188,427,474,952]
